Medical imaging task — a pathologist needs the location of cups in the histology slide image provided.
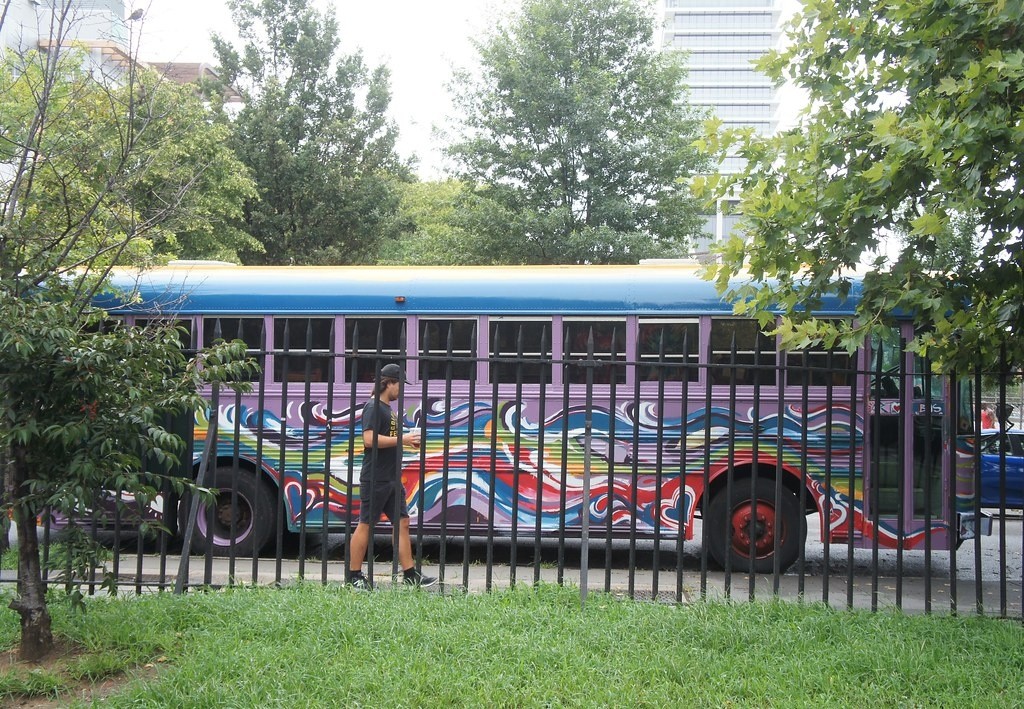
[409,428,421,449]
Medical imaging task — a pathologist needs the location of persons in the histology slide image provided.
[981,401,995,429]
[349,363,438,591]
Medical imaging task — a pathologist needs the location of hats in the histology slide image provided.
[381,363,412,385]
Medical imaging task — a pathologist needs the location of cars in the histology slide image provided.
[980,426,1024,509]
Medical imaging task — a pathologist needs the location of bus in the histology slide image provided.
[0,254,996,575]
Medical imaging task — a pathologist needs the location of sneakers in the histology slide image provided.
[348,574,379,596]
[402,571,438,589]
